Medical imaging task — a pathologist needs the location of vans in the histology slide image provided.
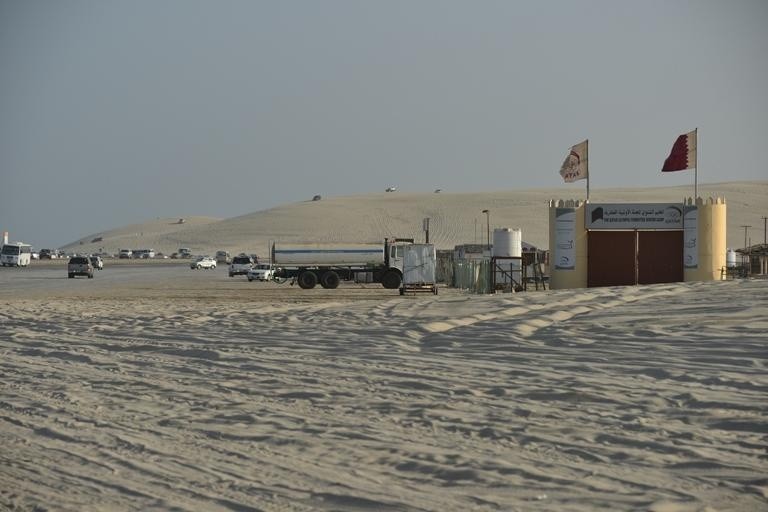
[216,251,231,263]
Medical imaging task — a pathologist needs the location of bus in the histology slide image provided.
[1,243,33,267]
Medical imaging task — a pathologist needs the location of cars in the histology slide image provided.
[247,263,277,282]
[178,218,186,223]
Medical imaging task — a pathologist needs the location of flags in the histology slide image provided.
[560,139,588,183]
[661,128,697,172]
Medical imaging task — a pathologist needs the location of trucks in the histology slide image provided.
[270,237,416,289]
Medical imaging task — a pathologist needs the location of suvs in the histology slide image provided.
[90,256,103,270]
[192,255,217,271]
[32,249,193,260]
[230,255,257,277]
[67,256,94,279]
[385,187,396,192]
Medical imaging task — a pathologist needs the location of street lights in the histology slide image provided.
[482,209,491,245]
